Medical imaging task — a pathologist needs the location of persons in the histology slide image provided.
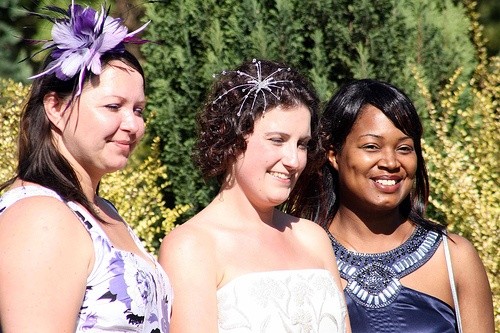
[0,46,174,333]
[159,60,353,333]
[303,77,495,333]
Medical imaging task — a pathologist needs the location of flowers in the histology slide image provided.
[5,0,162,138]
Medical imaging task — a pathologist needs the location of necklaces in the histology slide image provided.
[86,193,101,213]
